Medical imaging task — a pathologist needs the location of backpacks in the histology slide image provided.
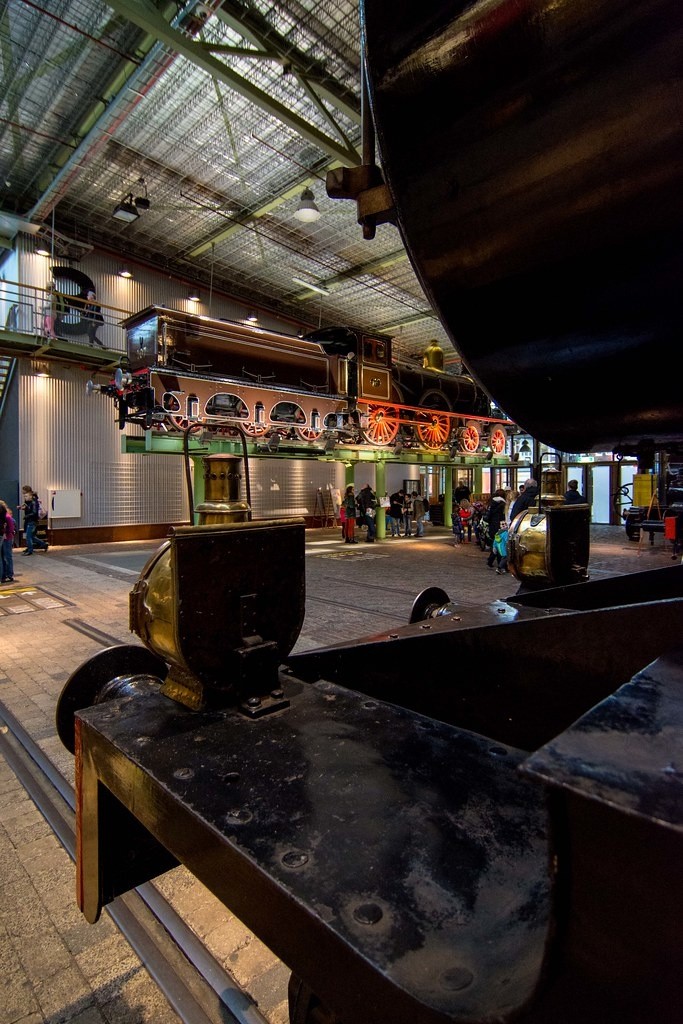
[37,499,47,520]
[420,499,429,512]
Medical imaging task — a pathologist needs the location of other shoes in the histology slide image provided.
[23,543,48,556]
[342,529,516,578]
[0,576,14,584]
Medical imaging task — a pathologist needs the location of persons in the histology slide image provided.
[411,491,425,537]
[21,492,49,556]
[83,291,109,350]
[563,479,588,504]
[338,486,391,545]
[44,281,65,340]
[458,481,525,575]
[450,507,465,549]
[510,478,539,523]
[454,481,471,505]
[38,285,56,339]
[389,489,405,537]
[0,500,18,585]
[16,486,43,552]
[402,494,413,537]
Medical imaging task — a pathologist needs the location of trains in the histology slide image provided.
[85,304,516,454]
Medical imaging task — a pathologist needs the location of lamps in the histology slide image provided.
[112,174,140,224]
[33,236,329,339]
[294,183,322,224]
